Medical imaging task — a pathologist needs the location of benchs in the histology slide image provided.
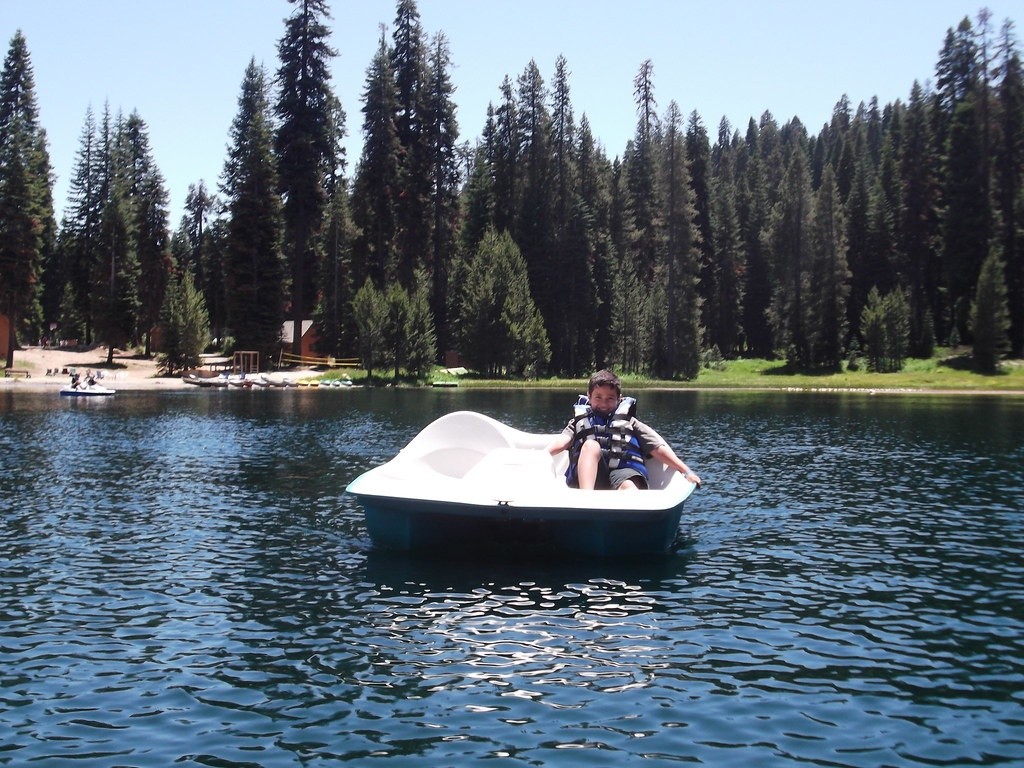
[5,367,32,378]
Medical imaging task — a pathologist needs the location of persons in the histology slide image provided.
[41,337,47,349]
[544,363,703,490]
[70,368,96,389]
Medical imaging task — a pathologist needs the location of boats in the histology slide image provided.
[181,374,364,390]
[344,409,699,567]
[60,377,116,397]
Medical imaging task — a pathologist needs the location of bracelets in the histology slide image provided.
[683,469,694,478]
